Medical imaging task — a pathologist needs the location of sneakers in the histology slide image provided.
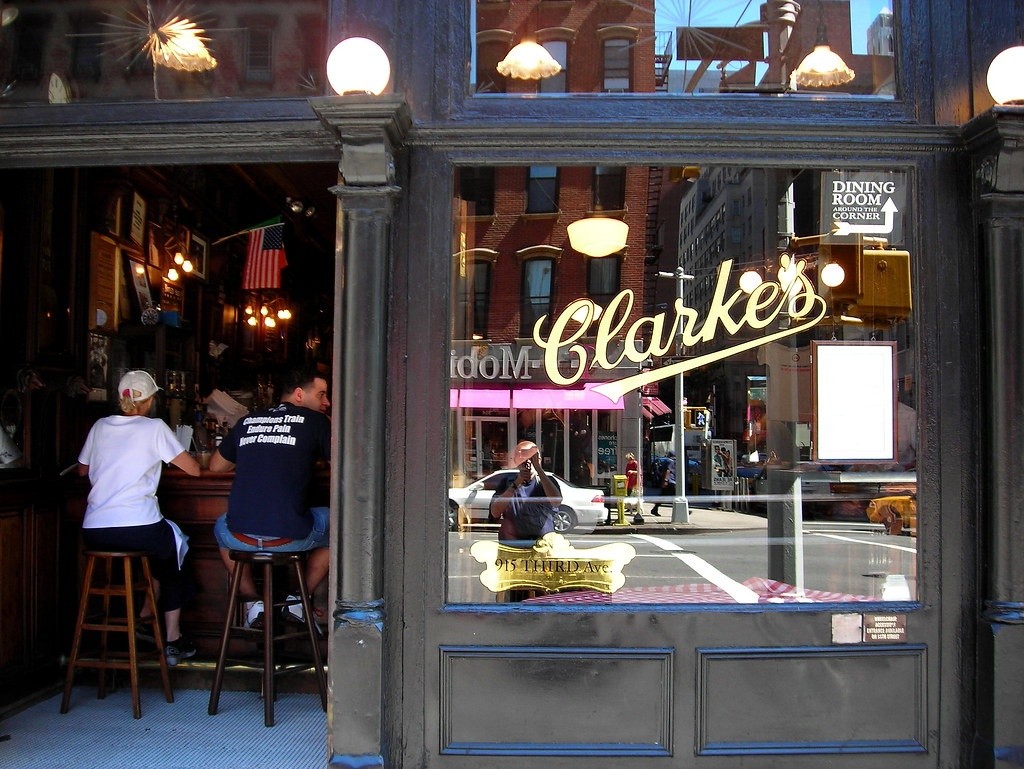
[282,594,324,635]
[243,602,265,629]
[165,634,198,665]
[134,623,159,642]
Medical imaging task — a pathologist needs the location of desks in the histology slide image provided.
[522,576,885,603]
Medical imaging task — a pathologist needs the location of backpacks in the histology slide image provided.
[651,460,665,486]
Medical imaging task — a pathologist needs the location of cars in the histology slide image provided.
[443,466,611,536]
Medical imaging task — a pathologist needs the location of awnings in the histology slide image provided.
[513,382,624,410]
[641,396,671,419]
[449,382,510,409]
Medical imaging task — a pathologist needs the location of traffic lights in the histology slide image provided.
[684,405,709,432]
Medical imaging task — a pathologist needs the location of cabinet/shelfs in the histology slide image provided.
[117,319,202,471]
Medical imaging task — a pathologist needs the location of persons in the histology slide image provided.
[78,371,199,665]
[713,446,733,477]
[488,440,563,603]
[623,452,642,512]
[210,371,331,635]
[650,459,692,517]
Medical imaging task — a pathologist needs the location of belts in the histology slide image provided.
[233,530,297,548]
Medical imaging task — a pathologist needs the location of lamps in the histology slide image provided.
[566,173,630,258]
[795,0,856,87]
[153,34,218,72]
[496,33,563,80]
[284,196,316,217]
[325,36,391,95]
[987,45,1024,106]
[260,297,292,327]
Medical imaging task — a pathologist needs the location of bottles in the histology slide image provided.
[254,375,267,408]
[195,411,203,427]
[205,414,218,435]
[263,375,274,408]
[222,422,228,436]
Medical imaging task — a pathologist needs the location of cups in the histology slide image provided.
[195,452,211,468]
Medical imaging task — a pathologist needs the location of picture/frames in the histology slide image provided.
[148,221,165,272]
[104,190,121,242]
[120,247,155,315]
[88,328,111,409]
[808,339,900,466]
[185,227,210,285]
[128,186,148,250]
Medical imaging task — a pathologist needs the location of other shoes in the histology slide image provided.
[651,510,661,516]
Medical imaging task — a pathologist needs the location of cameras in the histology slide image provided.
[524,462,531,472]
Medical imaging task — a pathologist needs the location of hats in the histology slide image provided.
[118,369,164,401]
[669,450,677,458]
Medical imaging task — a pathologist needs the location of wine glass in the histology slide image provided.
[208,425,224,451]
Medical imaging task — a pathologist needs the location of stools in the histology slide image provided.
[59,547,175,720]
[208,548,327,728]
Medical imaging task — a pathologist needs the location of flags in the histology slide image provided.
[242,214,288,290]
